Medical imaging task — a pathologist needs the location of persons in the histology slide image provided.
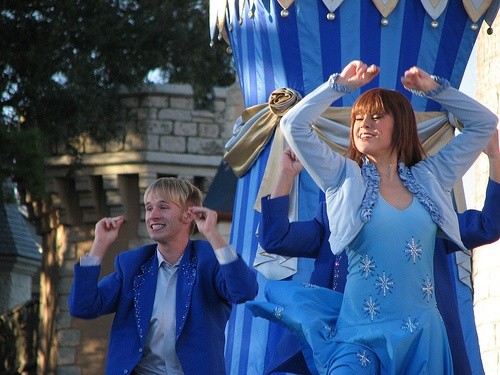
[257,126,500,375]
[281,59,500,374]
[68,176,260,375]
